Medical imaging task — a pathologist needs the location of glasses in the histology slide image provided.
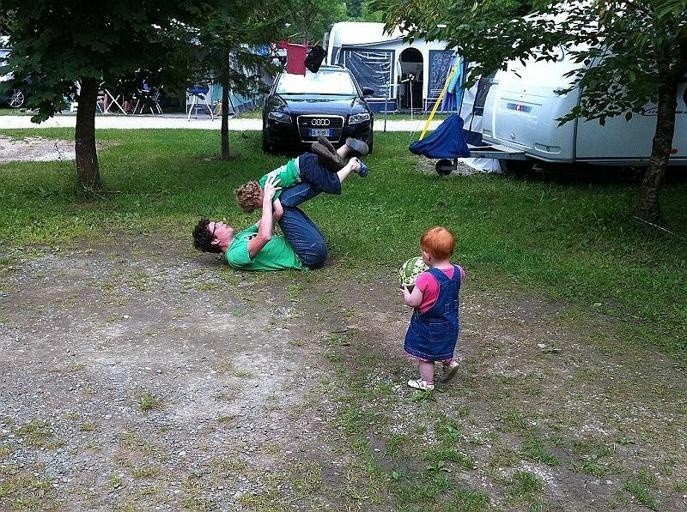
[212,222,218,237]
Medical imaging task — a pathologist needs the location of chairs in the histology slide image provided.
[71,75,214,121]
[425,88,442,112]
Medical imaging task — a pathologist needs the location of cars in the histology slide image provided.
[0,47,46,111]
[259,64,377,156]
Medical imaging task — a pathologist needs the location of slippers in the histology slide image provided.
[345,130,370,178]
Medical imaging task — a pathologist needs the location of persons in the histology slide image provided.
[192,135,345,271]
[235,137,369,221]
[400,226,466,391]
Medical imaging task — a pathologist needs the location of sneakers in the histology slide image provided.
[441,361,459,383]
[408,378,435,391]
[311,135,348,171]
